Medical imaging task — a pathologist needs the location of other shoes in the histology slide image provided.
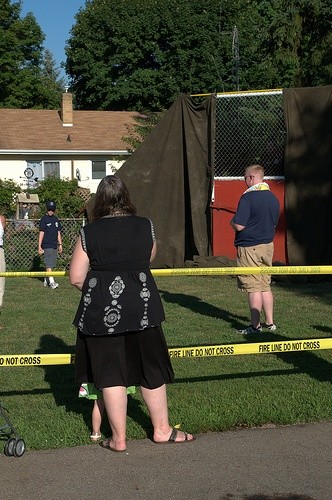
[49,282,58,289]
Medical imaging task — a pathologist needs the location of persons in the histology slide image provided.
[69,175,196,452]
[79,383,135,443]
[39,201,62,290]
[231,164,280,334]
[0,215,6,306]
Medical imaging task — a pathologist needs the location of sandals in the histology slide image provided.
[90,432,101,441]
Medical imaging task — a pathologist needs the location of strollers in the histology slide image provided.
[0,402,26,458]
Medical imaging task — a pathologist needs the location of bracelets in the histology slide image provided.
[59,244,62,245]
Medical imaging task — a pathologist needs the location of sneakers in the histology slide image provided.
[262,322,276,330]
[239,325,262,334]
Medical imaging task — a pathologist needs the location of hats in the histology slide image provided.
[46,201,56,207]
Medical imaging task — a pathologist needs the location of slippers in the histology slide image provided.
[99,437,126,452]
[155,428,196,444]
